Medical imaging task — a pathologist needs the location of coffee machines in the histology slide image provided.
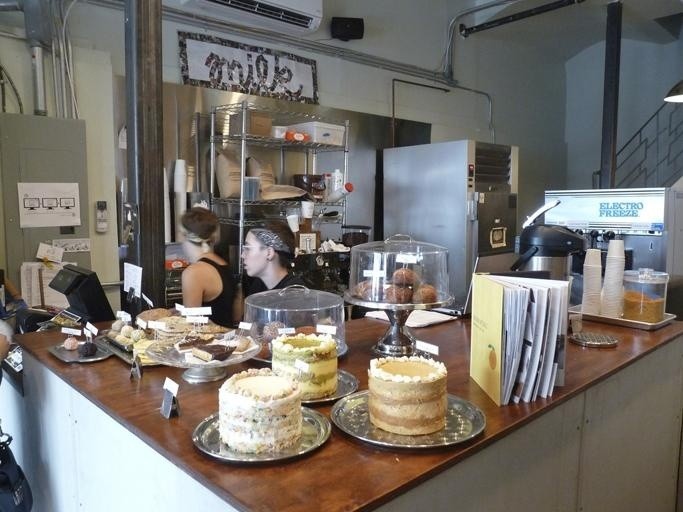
[339,225,372,288]
[543,189,682,305]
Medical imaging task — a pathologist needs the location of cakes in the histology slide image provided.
[270,333,337,399]
[366,355,448,435]
[178,330,215,349]
[192,345,237,362]
[216,367,302,453]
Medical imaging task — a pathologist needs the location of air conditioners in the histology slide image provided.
[181,0,323,34]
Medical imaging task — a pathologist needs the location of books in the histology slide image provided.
[469,269,571,408]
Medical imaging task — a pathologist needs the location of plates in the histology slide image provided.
[301,368,359,405]
[330,389,487,449]
[191,405,331,463]
[48,339,112,364]
[251,342,346,366]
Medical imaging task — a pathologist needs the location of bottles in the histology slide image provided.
[311,169,354,203]
[622,266,668,326]
[305,238,312,254]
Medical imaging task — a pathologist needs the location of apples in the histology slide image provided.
[488,344,496,369]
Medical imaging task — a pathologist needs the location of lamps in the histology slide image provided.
[664,78,683,103]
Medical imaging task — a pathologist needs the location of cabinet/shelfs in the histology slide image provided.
[208,104,351,282]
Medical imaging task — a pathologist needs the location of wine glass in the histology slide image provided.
[144,333,262,383]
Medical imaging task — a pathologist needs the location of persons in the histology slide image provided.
[176,209,244,329]
[0,271,33,511]
[239,218,308,292]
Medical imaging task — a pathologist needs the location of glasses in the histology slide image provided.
[241,244,267,252]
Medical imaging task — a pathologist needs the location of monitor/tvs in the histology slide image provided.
[48,264,116,323]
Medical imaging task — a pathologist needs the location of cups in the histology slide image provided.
[301,201,314,219]
[286,215,299,233]
[579,238,624,317]
[243,177,260,200]
[160,158,195,245]
[271,126,287,139]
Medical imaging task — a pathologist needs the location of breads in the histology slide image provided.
[254,336,269,359]
[109,307,232,344]
[353,268,437,304]
[287,325,320,337]
[263,321,286,338]
[233,335,250,352]
